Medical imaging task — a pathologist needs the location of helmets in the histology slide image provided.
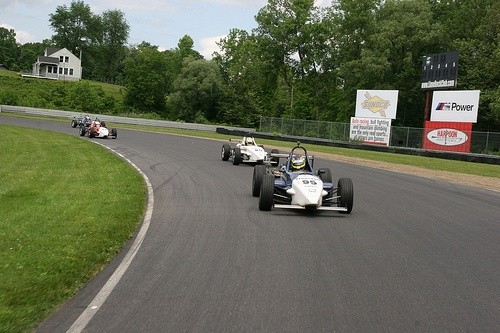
[246,137,252,146]
[291,156,306,172]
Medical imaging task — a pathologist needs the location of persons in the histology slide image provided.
[86,117,90,120]
[96,122,101,127]
[246,139,253,146]
[291,157,306,172]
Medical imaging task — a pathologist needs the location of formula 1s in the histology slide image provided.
[220,131,280,167]
[80,117,118,140]
[71,115,93,128]
[252,141,354,215]
